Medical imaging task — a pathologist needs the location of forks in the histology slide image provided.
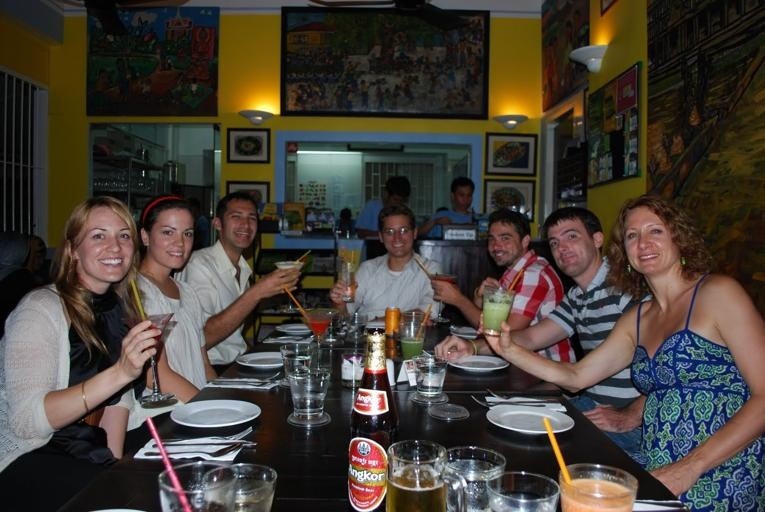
[155,426,259,440]
[482,384,561,401]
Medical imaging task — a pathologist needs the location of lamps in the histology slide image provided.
[495,114,528,128]
[567,44,608,73]
[239,111,273,125]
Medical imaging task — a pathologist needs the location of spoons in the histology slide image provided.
[143,443,242,457]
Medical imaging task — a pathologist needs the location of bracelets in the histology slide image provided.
[468,339,477,355]
[474,288,481,300]
[81,380,92,413]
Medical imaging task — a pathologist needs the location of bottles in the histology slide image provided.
[384,306,401,338]
[346,328,401,511]
[340,313,366,388]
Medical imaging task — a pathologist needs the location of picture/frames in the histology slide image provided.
[226,181,270,215]
[484,179,536,222]
[599,0,616,15]
[87,5,218,118]
[587,62,643,188]
[280,7,489,120]
[554,109,589,206]
[540,0,592,112]
[485,134,537,176]
[227,128,270,164]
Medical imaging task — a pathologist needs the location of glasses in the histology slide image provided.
[382,226,410,235]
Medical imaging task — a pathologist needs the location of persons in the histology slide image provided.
[418,177,474,238]
[284,14,484,115]
[330,205,446,317]
[0,195,162,512]
[174,191,300,377]
[477,194,765,512]
[434,207,655,453]
[128,193,218,447]
[353,176,412,238]
[432,210,576,364]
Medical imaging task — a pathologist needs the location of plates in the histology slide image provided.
[274,261,304,269]
[170,399,260,428]
[450,327,481,340]
[486,404,574,436]
[237,352,283,369]
[275,324,312,335]
[449,355,508,373]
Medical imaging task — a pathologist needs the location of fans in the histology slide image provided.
[84,0,132,37]
[308,1,464,29]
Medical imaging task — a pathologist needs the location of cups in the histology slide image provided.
[560,464,638,512]
[122,313,178,402]
[341,262,355,301]
[343,311,370,351]
[289,370,330,419]
[299,309,339,352]
[447,447,506,512]
[158,463,236,512]
[402,312,424,359]
[483,285,515,337]
[202,464,277,512]
[412,355,448,397]
[280,343,312,381]
[385,440,447,512]
[486,472,559,512]
